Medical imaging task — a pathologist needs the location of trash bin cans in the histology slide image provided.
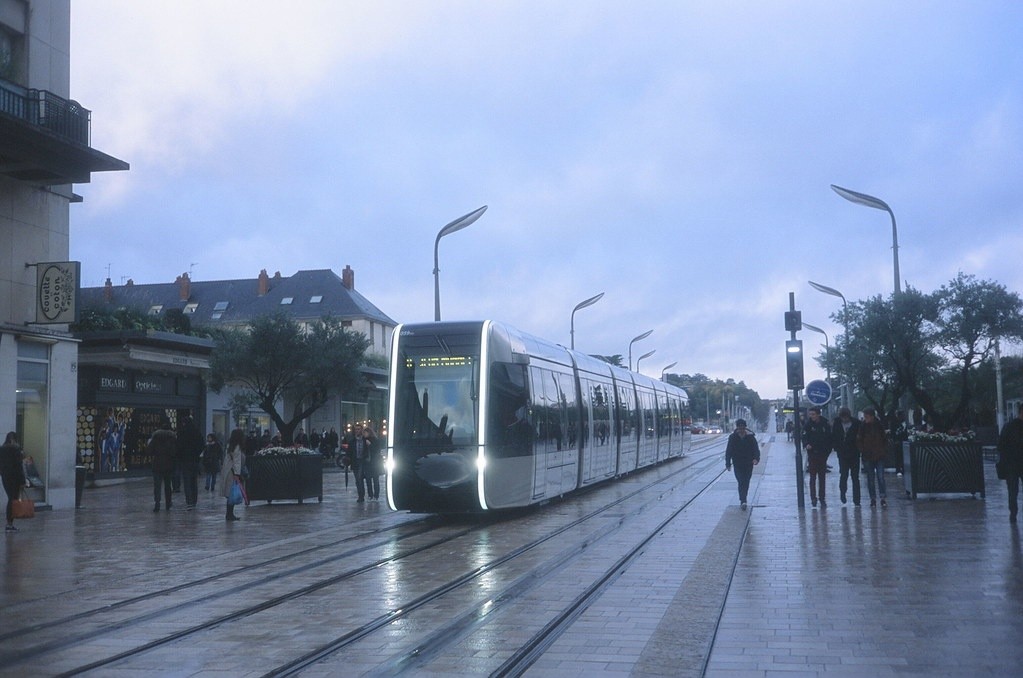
[75,466,87,507]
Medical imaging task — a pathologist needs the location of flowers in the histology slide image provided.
[906,426,977,443]
[254,441,319,456]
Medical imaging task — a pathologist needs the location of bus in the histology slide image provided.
[385,317,694,518]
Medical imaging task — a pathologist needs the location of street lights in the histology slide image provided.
[801,321,832,415]
[432,203,488,321]
[571,291,605,349]
[637,350,656,373]
[731,395,757,435]
[629,329,653,370]
[661,361,678,382]
[830,184,916,501]
[808,280,855,416]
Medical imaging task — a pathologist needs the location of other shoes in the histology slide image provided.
[881,500,885,505]
[166,502,173,510]
[205,486,209,490]
[153,502,160,513]
[187,504,192,511]
[870,500,876,506]
[740,503,747,510]
[5,526,20,532]
[374,498,378,500]
[368,497,373,502]
[356,496,364,502]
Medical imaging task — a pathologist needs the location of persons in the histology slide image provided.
[0,431,26,532]
[894,409,909,477]
[76,440,83,465]
[145,417,204,512]
[725,419,760,511]
[998,403,1023,521]
[786,419,793,441]
[246,429,270,455]
[832,408,862,508]
[539,421,610,451]
[857,408,886,507]
[99,408,125,473]
[220,429,245,521]
[346,424,381,502]
[294,426,338,462]
[203,434,223,491]
[802,406,834,506]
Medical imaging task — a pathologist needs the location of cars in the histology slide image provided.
[693,425,723,434]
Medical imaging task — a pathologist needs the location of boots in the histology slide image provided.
[226,511,240,520]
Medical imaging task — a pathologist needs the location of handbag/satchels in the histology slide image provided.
[12,486,34,518]
[996,462,1006,479]
[227,480,244,506]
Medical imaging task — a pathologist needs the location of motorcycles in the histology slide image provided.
[335,444,349,469]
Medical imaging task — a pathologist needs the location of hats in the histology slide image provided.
[838,406,851,415]
[863,404,876,416]
[736,418,747,427]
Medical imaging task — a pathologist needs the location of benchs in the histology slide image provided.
[983,445,997,461]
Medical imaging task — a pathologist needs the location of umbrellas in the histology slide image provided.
[345,461,348,491]
[231,468,250,505]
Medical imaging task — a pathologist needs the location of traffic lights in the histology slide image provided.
[787,312,798,328]
[788,341,802,390]
